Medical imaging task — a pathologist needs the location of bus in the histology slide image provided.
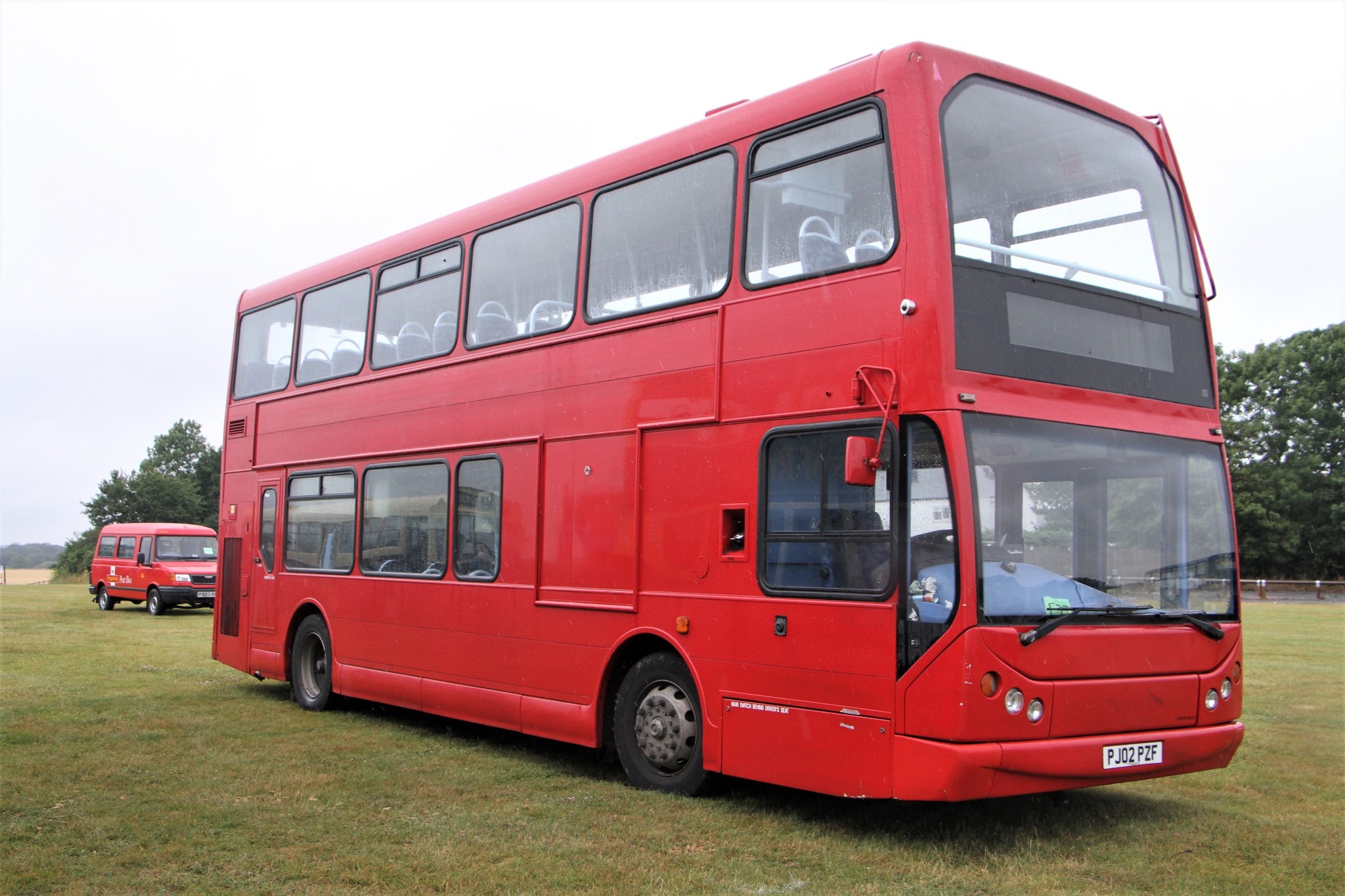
[212,41,1243,801]
[260,489,496,575]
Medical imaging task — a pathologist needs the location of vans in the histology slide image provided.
[89,523,218,615]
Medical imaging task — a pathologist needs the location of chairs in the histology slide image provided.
[797,215,891,279]
[273,299,629,386]
[371,527,496,579]
[820,507,884,590]
